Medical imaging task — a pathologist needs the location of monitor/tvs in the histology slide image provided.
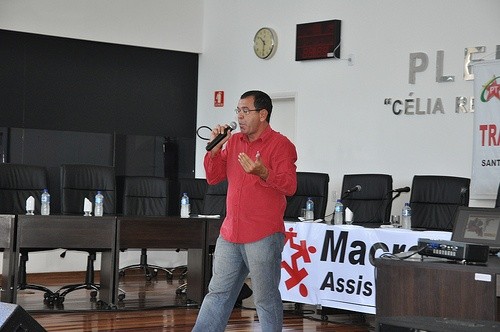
[450,206,500,255]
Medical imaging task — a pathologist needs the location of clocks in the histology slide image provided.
[254,28,279,59]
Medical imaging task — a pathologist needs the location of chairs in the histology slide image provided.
[120,176,176,281]
[204,180,229,215]
[53,164,126,303]
[172,178,204,279]
[408,175,471,231]
[283,172,329,220]
[331,173,394,228]
[0,162,57,306]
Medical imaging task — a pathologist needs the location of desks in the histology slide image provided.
[113,215,207,308]
[15,215,117,310]
[277,220,455,325]
[205,217,226,295]
[0,214,14,303]
[376,248,500,332]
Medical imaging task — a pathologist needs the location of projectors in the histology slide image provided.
[417,239,489,262]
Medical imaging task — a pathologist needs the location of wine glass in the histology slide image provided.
[26,200,35,215]
[345,211,355,225]
[83,202,92,216]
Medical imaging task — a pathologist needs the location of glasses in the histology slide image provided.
[234,108,261,116]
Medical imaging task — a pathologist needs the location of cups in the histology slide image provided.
[301,208,306,218]
[392,215,400,226]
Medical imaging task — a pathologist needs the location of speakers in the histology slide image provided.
[0,302,47,332]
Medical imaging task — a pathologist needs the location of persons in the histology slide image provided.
[190,90,298,332]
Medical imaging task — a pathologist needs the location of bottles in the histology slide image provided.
[334,199,344,225]
[402,202,412,229]
[94,191,104,216]
[306,198,314,220]
[41,189,50,215]
[181,193,190,218]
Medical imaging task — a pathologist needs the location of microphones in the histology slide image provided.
[344,184,361,193]
[205,121,237,151]
[389,186,410,192]
